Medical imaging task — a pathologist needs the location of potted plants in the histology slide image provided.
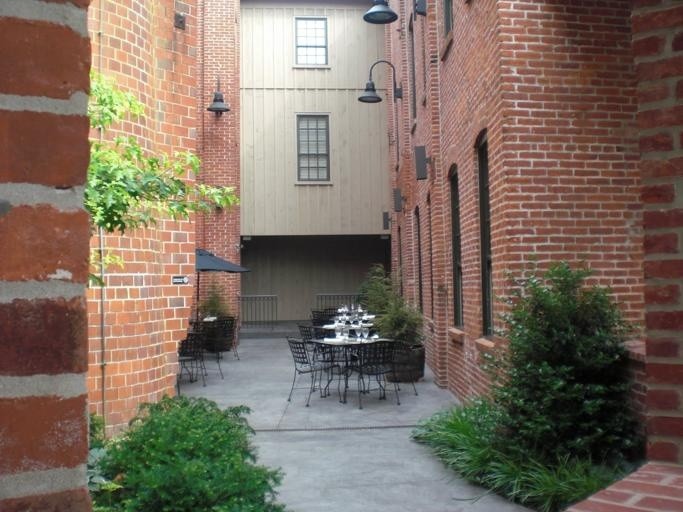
[374,303,425,382]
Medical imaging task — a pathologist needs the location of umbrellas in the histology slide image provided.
[196,248,252,318]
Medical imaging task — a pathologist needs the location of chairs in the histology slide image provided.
[178,314,239,386]
[286,306,418,409]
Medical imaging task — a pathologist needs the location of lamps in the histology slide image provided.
[364,0,397,24]
[358,61,403,102]
[207,74,230,116]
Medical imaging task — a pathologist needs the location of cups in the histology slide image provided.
[335,328,349,337]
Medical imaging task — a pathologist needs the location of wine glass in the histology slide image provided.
[355,327,369,338]
